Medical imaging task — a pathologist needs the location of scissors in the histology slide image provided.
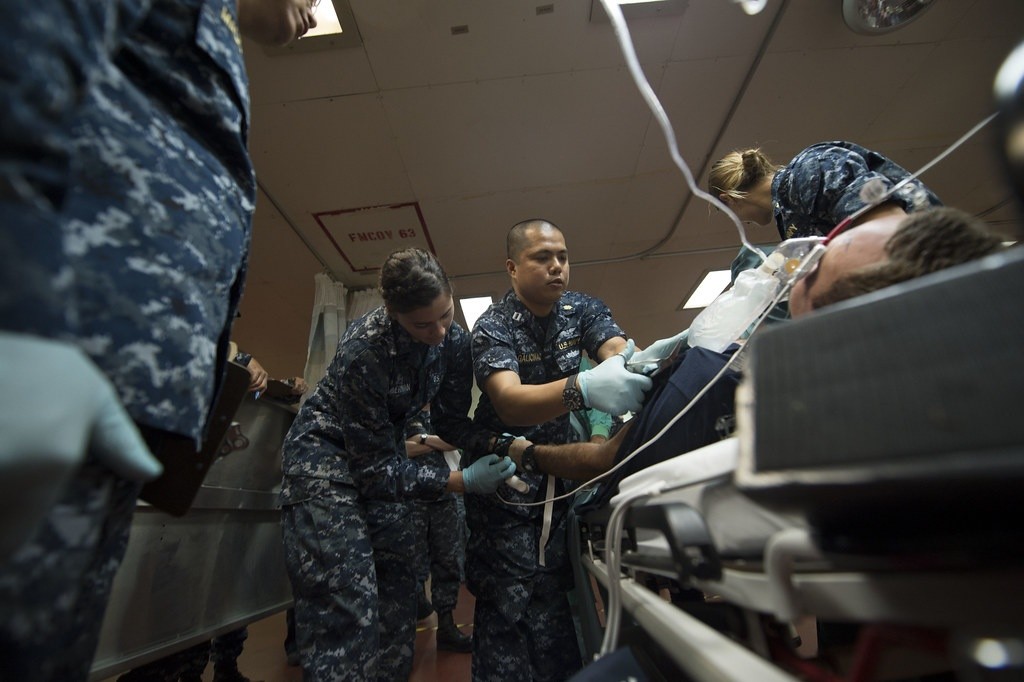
[617,338,688,379]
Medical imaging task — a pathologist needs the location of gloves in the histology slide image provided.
[462,453,516,495]
[0,333,166,563]
[577,338,652,416]
[626,328,689,376]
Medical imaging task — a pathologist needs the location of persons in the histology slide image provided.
[0,0,319,681]
[116,138,1008,682]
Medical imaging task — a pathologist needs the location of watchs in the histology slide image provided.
[562,373,591,412]
[522,445,537,472]
[419,433,427,444]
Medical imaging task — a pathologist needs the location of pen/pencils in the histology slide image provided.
[254,390,260,400]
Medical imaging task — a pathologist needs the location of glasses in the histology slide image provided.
[306,0,324,17]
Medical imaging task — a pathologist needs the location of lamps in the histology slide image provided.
[841,0,937,35]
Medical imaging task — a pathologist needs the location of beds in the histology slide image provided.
[567,243,1024,682]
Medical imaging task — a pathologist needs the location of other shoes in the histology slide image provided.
[213,658,251,682]
[287,649,300,666]
[436,624,473,654]
[414,600,432,624]
